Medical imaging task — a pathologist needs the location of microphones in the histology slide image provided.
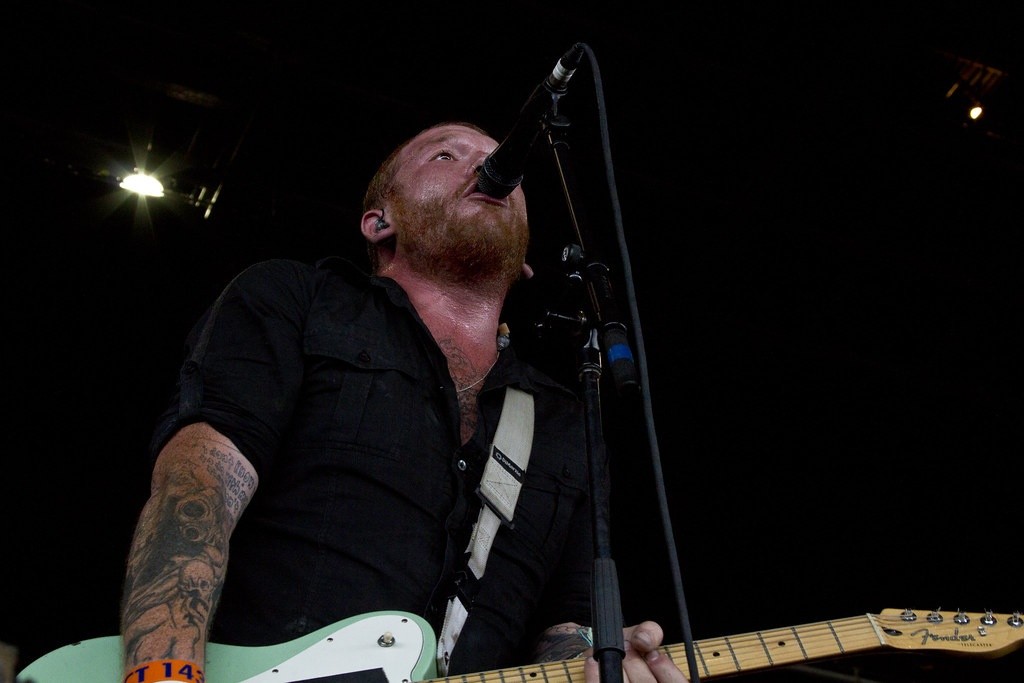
[587,248,643,399]
[475,42,584,200]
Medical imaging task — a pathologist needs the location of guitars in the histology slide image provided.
[9,605,1024,683]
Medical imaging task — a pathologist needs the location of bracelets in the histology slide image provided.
[123,659,204,683]
[568,649,586,659]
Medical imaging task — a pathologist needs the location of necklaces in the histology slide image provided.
[457,351,500,394]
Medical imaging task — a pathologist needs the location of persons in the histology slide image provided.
[122,123,691,683]
